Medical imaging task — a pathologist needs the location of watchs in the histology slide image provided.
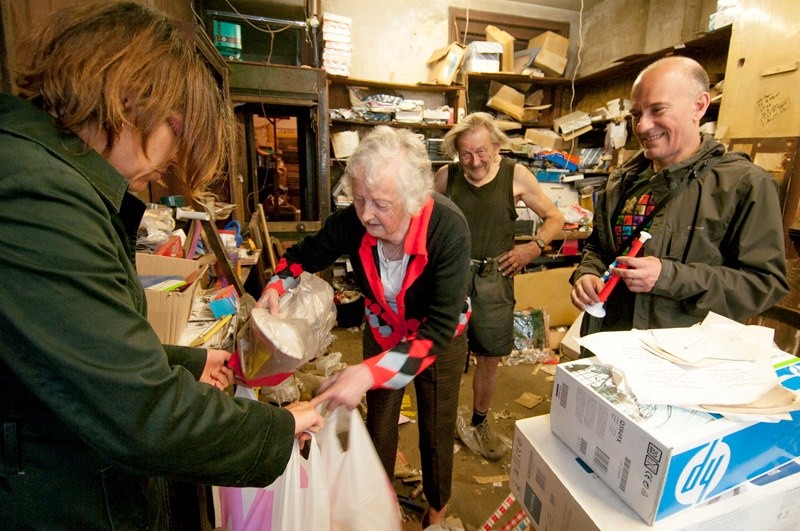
[534,239,546,251]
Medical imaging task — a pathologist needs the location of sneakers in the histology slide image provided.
[473,418,502,460]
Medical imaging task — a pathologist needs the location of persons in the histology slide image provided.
[431,113,566,463]
[569,55,793,358]
[255,125,472,529]
[0,0,323,531]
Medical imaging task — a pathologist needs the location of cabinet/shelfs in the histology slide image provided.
[326,73,467,164]
[453,22,574,265]
[574,22,732,145]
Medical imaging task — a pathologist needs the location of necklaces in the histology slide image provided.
[382,243,404,263]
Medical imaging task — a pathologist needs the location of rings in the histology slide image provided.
[630,278,634,286]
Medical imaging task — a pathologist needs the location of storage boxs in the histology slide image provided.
[421,23,593,184]
[509,348,800,531]
[133,252,217,346]
[330,129,360,160]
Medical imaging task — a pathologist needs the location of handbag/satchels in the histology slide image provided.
[317,402,403,531]
[215,427,329,530]
